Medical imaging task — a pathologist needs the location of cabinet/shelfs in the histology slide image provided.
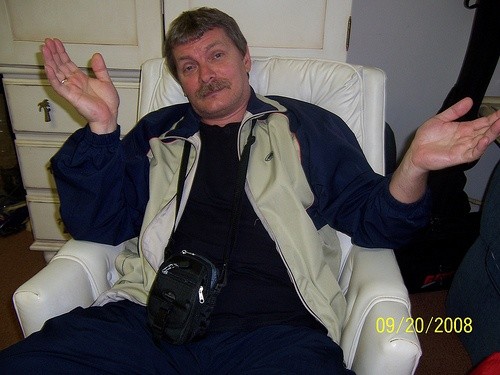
[0,0,353,266]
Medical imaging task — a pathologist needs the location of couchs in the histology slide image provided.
[12,56,422,375]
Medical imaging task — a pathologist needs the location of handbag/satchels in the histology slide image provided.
[146,249,221,343]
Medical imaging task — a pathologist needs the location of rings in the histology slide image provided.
[61,76,68,84]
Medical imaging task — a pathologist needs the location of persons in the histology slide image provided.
[0,6,499,375]
